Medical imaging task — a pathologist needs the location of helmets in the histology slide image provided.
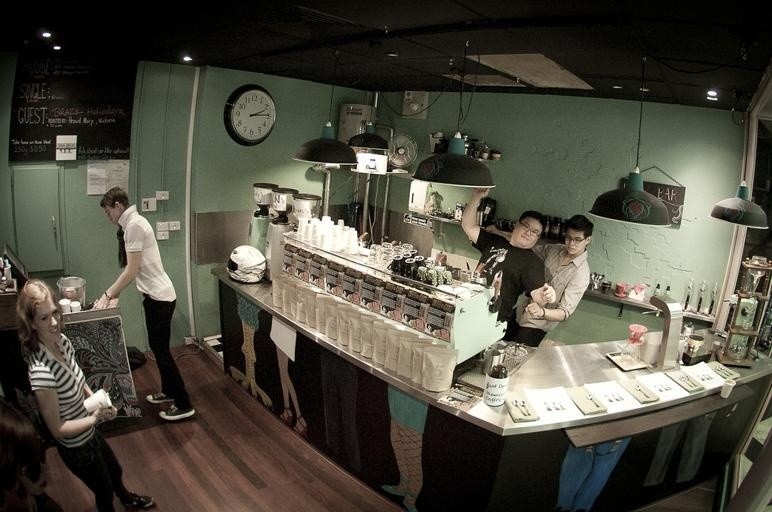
[226,244,267,284]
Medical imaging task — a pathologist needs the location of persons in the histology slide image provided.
[461,187,558,341]
[235,290,263,397]
[642,412,719,488]
[319,342,362,477]
[270,315,308,435]
[15,278,155,512]
[554,437,634,512]
[379,379,429,512]
[485,214,593,347]
[0,396,63,512]
[89,186,196,421]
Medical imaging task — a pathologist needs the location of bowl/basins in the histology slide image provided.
[491,153,501,160]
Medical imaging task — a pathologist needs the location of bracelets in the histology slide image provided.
[103,290,113,302]
[469,197,481,208]
[540,307,550,321]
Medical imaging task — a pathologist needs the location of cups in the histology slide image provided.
[602,281,612,295]
[627,324,648,349]
[58,298,81,314]
[81,388,112,413]
[480,343,507,376]
[616,282,629,295]
[481,153,488,159]
[369,241,454,287]
[720,378,737,399]
[639,332,662,364]
[296,216,360,252]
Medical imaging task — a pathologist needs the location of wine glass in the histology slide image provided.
[748,256,768,297]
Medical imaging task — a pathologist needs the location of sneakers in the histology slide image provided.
[147,391,175,405]
[158,405,196,421]
[123,495,155,510]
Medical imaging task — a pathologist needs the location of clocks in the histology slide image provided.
[224,80,277,147]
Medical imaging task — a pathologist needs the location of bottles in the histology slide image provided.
[481,365,510,407]
[688,335,704,354]
[653,284,672,297]
[541,215,571,242]
[682,321,694,339]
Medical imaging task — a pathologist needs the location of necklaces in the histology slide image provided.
[39,340,65,364]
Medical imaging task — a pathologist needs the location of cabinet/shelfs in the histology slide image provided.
[11,164,64,270]
[716,255,771,368]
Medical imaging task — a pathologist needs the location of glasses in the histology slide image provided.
[520,219,541,237]
[567,236,590,244]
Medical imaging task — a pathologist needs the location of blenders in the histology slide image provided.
[248,182,322,281]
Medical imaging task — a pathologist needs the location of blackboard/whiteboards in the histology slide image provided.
[620,177,686,225]
[8,43,140,163]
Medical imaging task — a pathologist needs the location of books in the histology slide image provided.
[436,381,485,413]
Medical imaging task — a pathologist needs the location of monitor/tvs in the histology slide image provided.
[2,240,28,289]
